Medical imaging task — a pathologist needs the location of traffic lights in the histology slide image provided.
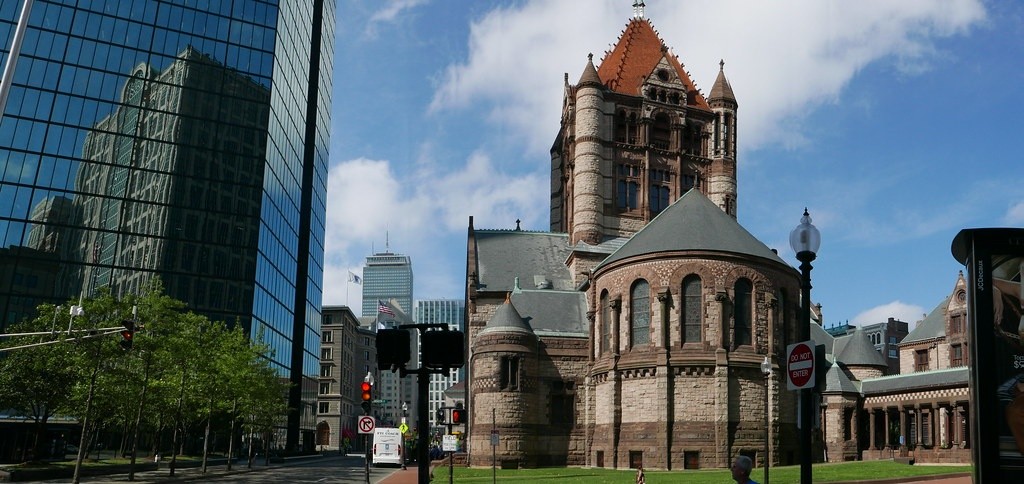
[438,410,445,423]
[360,381,371,413]
[453,410,460,423]
[120,319,134,351]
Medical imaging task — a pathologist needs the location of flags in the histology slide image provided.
[347,270,362,286]
[377,299,396,318]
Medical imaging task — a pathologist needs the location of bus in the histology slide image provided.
[372,427,403,468]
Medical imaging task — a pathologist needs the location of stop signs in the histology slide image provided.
[786,340,816,391]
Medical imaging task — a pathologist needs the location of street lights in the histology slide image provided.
[789,207,822,484]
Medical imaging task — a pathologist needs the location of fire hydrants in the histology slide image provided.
[636,465,645,484]
